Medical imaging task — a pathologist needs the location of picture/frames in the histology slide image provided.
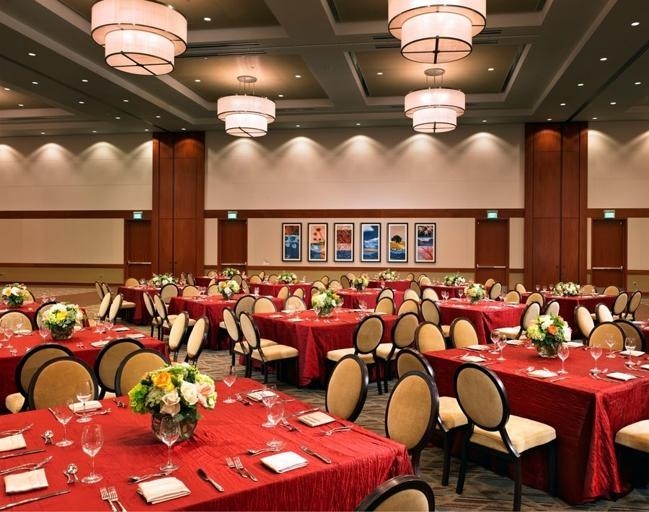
[387,223,408,263]
[334,223,354,262]
[360,223,381,262]
[282,223,302,262]
[308,223,328,262]
[415,223,436,263]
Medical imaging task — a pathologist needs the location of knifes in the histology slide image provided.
[1,488,71,510]
[0,449,48,459]
[197,468,224,491]
[285,406,319,419]
[300,445,331,464]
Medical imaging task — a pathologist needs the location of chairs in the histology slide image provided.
[0,270,649,512]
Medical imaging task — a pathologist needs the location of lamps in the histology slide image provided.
[217,76,276,138]
[90,0,188,76]
[388,0,486,64]
[403,69,465,133]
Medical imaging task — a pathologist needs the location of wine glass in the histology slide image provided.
[75,380,93,422]
[222,363,237,405]
[286,298,368,321]
[80,423,105,485]
[266,401,284,446]
[53,398,75,447]
[260,383,276,429]
[535,283,555,294]
[0,290,122,351]
[159,415,181,472]
[490,327,637,374]
[441,288,507,307]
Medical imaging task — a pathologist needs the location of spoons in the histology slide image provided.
[64,464,81,486]
[126,473,166,484]
[247,446,279,457]
[75,408,112,418]
[41,429,54,445]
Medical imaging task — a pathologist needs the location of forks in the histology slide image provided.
[281,415,299,430]
[0,456,52,476]
[225,456,258,482]
[0,422,33,437]
[320,426,351,436]
[100,485,129,512]
[235,392,253,406]
[111,398,129,409]
